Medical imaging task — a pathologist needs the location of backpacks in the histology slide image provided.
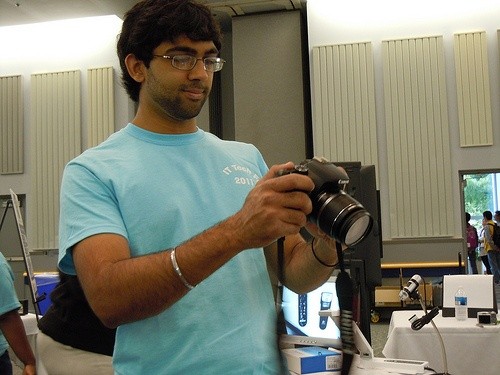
[486,223,500,248]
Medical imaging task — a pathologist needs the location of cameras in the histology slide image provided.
[276,157,373,247]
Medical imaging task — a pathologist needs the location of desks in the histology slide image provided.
[9,312,44,375]
[382,310,500,375]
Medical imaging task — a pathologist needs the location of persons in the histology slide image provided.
[478,210,500,284]
[55,1,349,375]
[37,269,117,375]
[0,252,36,375]
[465,212,479,275]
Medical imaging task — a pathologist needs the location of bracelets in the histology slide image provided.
[171,248,198,290]
[311,237,339,266]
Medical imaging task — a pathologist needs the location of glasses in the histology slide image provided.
[147,54,226,72]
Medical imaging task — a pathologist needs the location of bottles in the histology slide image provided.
[454,284,468,322]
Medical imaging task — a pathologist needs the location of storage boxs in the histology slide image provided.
[24,272,60,314]
[375,285,442,306]
[281,346,342,375]
